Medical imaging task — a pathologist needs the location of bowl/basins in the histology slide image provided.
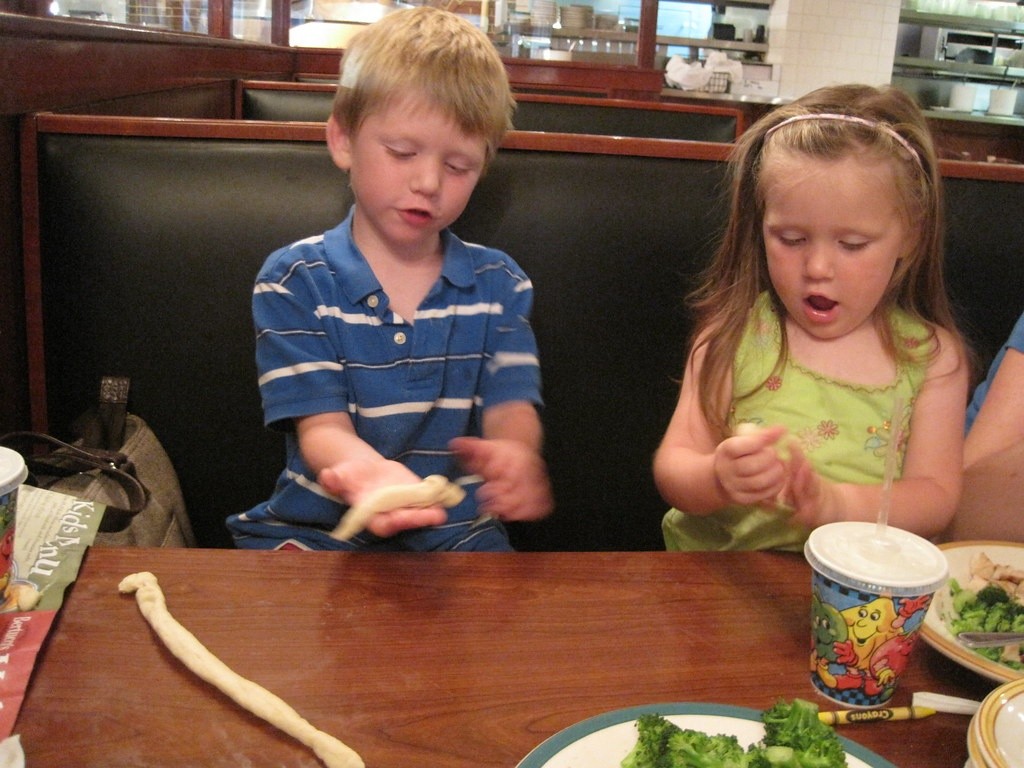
[544,49,573,61]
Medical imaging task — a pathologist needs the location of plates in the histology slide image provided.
[530,0,619,31]
[967,676,1024,768]
[917,538,1024,683]
[514,700,901,768]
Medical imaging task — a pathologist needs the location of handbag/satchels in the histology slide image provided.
[0,377,195,551]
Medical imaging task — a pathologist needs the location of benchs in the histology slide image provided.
[23,75,1023,554]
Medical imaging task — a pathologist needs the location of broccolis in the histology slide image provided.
[619,696,849,768]
[946,580,1024,670]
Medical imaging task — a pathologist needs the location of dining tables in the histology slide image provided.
[12,550,1001,767]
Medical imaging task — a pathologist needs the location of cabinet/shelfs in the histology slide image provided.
[507,0,1024,128]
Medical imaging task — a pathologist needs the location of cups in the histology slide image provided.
[949,83,976,112]
[987,88,1017,115]
[0,445,28,610]
[804,520,949,710]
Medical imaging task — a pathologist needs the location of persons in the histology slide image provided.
[938,311,1024,546]
[230,7,558,554]
[647,82,971,555]
